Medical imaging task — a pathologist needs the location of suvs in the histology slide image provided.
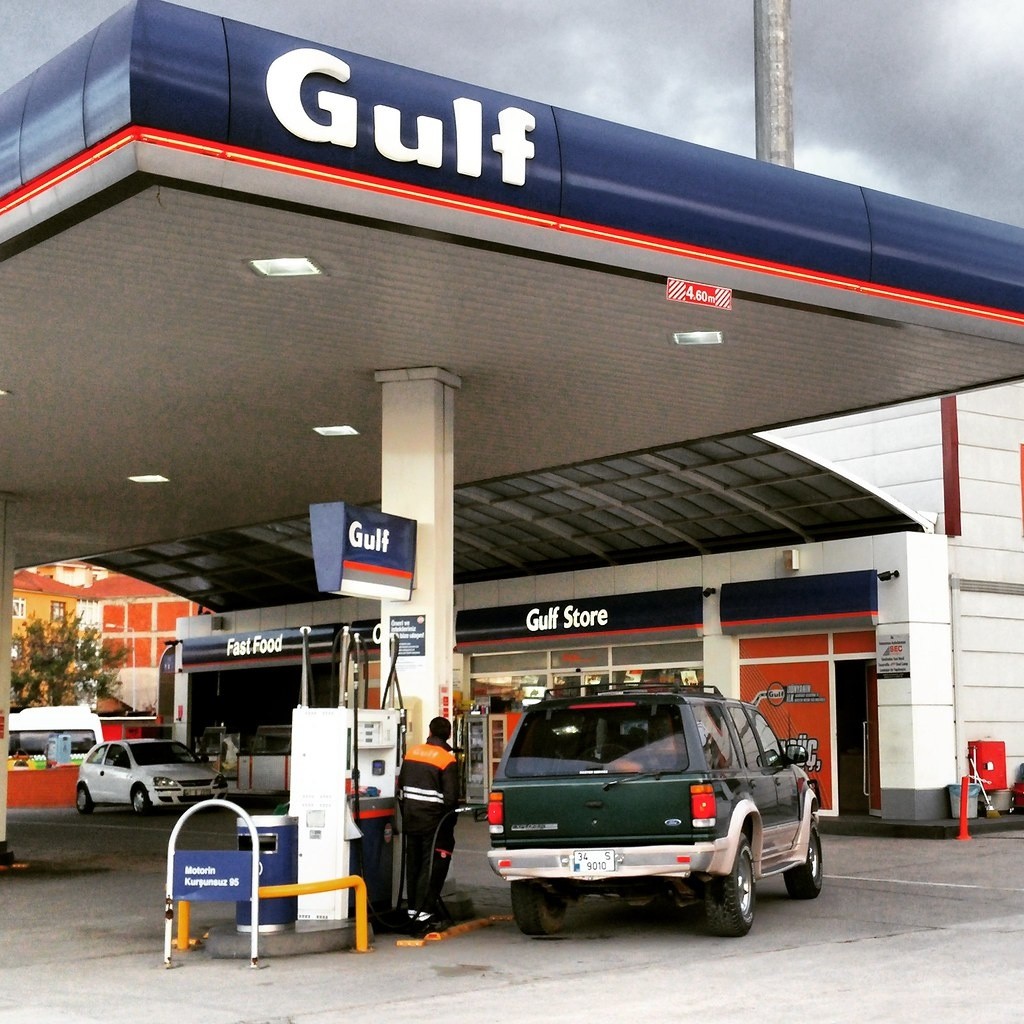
[487,679,825,940]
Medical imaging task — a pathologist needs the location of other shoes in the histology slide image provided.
[404,909,422,932]
[415,912,448,937]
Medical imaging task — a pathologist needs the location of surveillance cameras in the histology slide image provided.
[703,588,711,598]
[878,571,891,582]
[164,640,179,646]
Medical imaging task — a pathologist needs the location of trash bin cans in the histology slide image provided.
[234,815,299,932]
[948,783,980,819]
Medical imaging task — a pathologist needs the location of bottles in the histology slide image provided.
[34,755,46,769]
[71,754,87,764]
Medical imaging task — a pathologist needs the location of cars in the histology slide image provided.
[74,739,229,817]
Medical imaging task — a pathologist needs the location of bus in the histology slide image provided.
[8,705,104,770]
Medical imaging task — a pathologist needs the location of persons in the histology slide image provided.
[395,716,461,930]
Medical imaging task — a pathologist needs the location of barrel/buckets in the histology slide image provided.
[991,790,1011,810]
